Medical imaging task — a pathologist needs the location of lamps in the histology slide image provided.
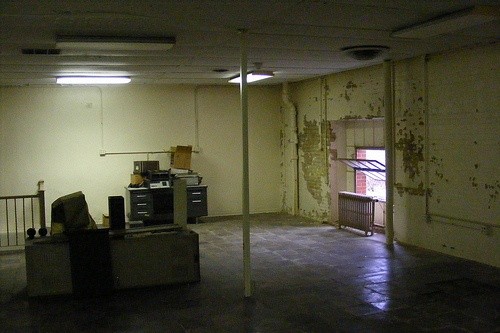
[341,44,390,62]
[56,77,132,85]
[55,34,177,49]
[229,70,274,85]
[391,5,500,41]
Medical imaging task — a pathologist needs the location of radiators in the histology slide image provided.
[338,191,378,236]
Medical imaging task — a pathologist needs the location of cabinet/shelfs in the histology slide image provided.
[125,184,209,223]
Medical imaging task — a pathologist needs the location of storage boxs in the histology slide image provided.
[130,143,197,188]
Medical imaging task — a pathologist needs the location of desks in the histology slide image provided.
[26,228,202,298]
